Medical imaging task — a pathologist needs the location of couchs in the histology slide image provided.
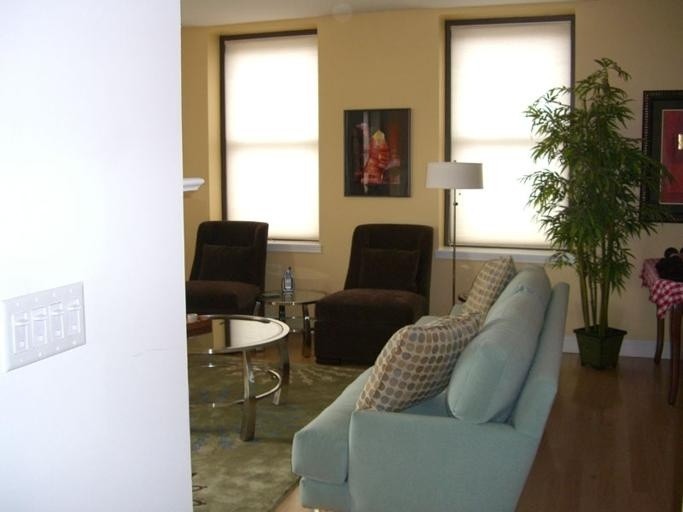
[286,258,571,511]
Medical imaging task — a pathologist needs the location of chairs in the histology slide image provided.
[185,220,270,322]
[313,222,435,367]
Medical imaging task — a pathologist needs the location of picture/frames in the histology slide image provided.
[342,106,413,201]
[638,88,683,224]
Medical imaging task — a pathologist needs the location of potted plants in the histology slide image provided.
[522,55,674,374]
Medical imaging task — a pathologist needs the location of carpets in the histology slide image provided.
[188,352,363,512]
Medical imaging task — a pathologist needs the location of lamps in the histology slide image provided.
[425,159,486,306]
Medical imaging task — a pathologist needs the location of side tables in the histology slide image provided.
[254,288,326,358]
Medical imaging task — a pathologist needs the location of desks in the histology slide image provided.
[642,255,681,405]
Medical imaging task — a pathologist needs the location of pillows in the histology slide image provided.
[353,255,517,418]
